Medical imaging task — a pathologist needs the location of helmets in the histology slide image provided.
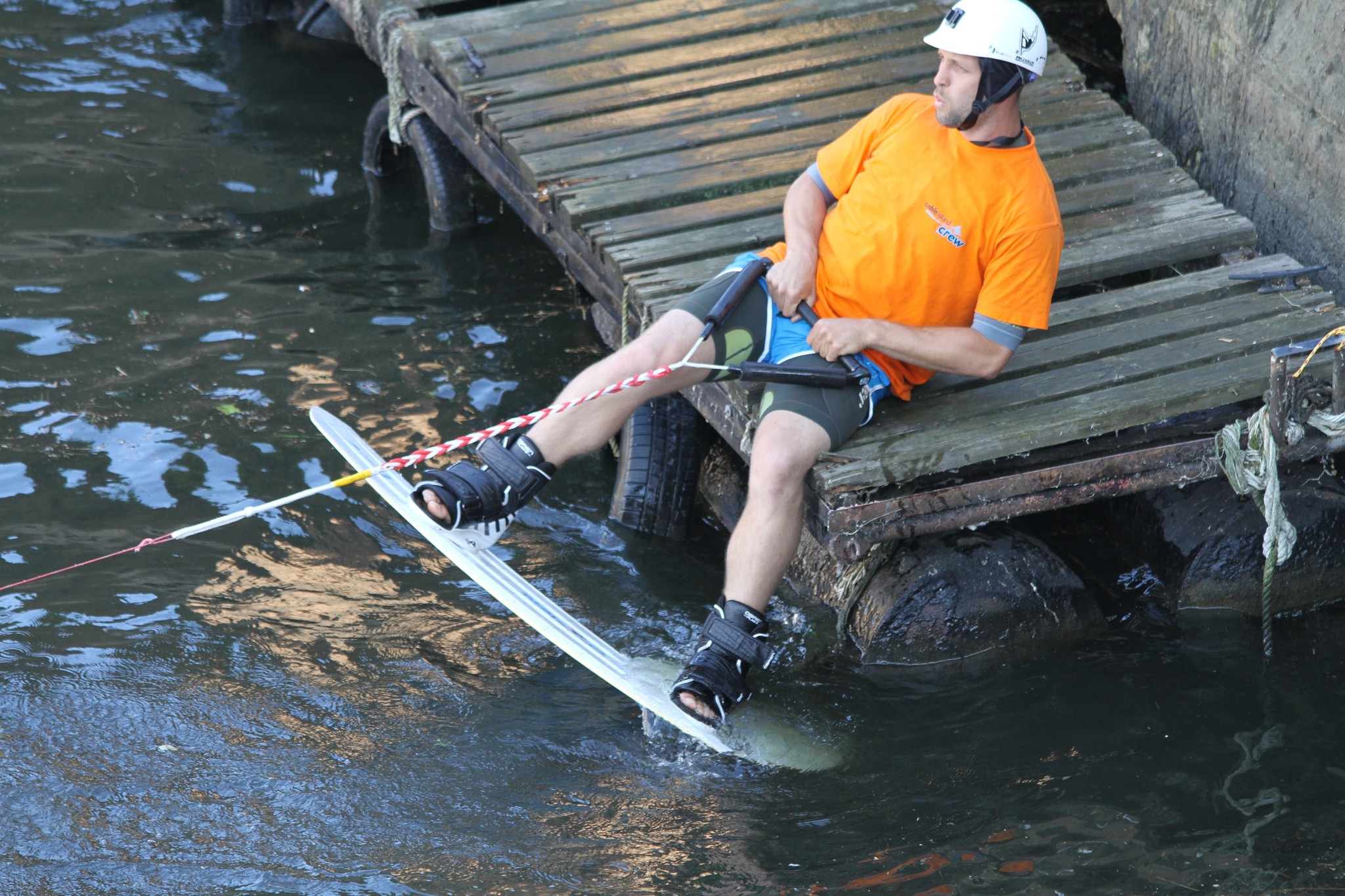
[923,0,1047,77]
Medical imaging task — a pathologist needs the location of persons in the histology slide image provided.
[409,0,1066,730]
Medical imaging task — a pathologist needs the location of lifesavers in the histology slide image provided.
[364,95,494,227]
[609,393,705,539]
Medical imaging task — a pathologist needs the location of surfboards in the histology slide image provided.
[306,404,723,756]
